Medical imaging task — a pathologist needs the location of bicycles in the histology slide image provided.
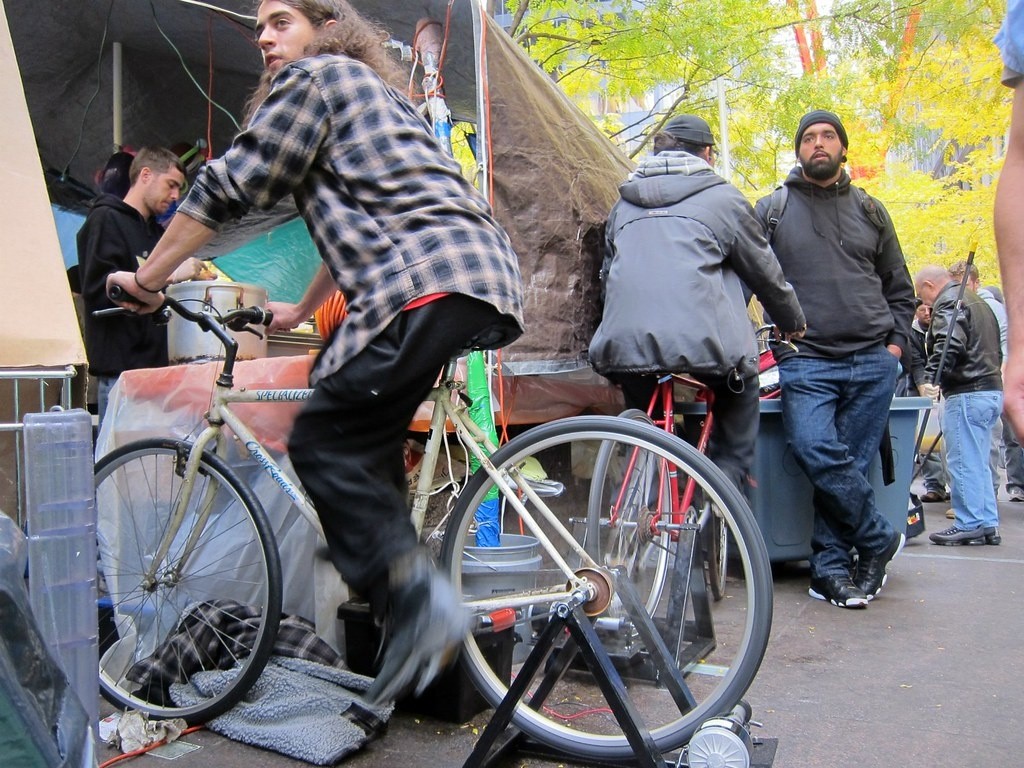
[568,324,806,641]
[65,283,774,762]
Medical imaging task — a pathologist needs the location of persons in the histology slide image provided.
[994,0,1024,448]
[76,145,217,435]
[739,110,916,607]
[895,260,1024,545]
[589,114,807,519]
[107,0,525,705]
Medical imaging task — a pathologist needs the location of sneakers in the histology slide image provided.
[1008,486,1024,501]
[851,528,905,601]
[984,526,1001,545]
[808,574,869,608]
[929,526,986,546]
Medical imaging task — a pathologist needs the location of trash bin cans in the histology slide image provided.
[664,395,935,565]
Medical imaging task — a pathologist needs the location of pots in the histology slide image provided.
[166,283,267,364]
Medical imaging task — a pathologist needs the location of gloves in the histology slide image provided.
[923,383,939,399]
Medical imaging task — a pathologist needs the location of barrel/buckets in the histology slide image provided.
[461,534,542,664]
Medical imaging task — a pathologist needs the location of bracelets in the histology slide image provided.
[134,273,162,293]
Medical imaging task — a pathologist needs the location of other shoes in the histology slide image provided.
[921,492,944,502]
[946,508,955,519]
[366,569,468,701]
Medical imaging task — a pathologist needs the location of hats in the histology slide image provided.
[915,297,922,308]
[663,114,715,147]
[795,109,848,159]
